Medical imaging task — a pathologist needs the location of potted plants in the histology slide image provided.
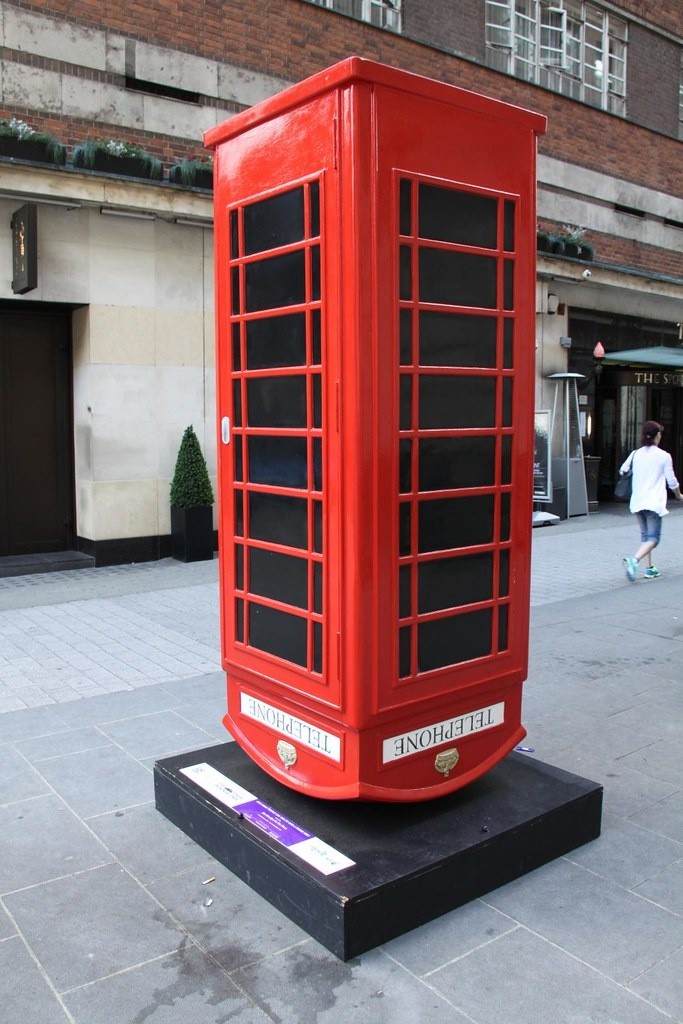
[169,423,215,563]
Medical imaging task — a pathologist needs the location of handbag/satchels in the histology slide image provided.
[615,450,636,499]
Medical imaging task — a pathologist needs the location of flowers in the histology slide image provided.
[536,223,588,254]
[0,118,67,169]
[171,153,213,187]
[71,135,161,182]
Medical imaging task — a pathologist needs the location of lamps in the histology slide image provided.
[591,340,604,377]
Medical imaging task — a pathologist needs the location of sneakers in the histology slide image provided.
[623,557,638,581]
[644,565,660,578]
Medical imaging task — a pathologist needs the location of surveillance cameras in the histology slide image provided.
[583,270,591,279]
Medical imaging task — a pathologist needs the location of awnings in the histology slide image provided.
[601,345,683,375]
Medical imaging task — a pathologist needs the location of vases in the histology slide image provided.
[169,167,213,190]
[537,236,594,262]
[0,135,66,166]
[73,150,163,181]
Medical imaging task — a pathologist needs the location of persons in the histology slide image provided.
[619,421,683,581]
